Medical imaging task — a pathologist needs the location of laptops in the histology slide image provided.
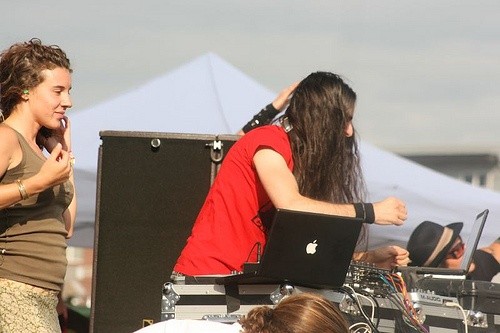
[186,208,363,288]
[395,209,488,275]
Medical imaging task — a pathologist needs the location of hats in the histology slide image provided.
[407,221,463,274]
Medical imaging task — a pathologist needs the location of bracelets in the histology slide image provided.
[16,177,29,200]
[353,202,375,224]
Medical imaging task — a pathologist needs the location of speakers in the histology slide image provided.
[89,130,243,333]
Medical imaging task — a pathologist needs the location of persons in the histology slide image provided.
[169,72,407,278]
[236,81,299,135]
[237,293,351,333]
[0,37,76,333]
[406,220,500,282]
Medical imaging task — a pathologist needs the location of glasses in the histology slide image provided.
[443,243,464,259]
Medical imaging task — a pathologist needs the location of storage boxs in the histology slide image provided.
[160,284,476,332]
